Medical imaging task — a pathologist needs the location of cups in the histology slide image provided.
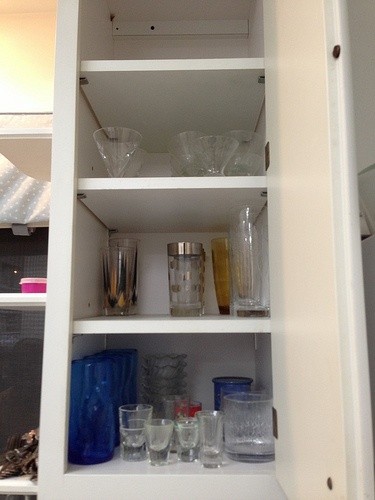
[74,359,116,464]
[148,417,174,465]
[226,130,265,174]
[120,404,153,460]
[122,419,146,460]
[227,392,273,464]
[212,237,249,314]
[72,349,138,464]
[198,411,225,469]
[97,245,130,315]
[174,419,197,461]
[110,235,141,314]
[167,242,207,316]
[161,395,203,417]
[212,376,253,409]
[228,201,270,316]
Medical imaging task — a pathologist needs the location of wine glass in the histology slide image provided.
[92,126,144,176]
[190,135,238,176]
[167,132,210,176]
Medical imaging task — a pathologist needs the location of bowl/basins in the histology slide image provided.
[142,353,190,408]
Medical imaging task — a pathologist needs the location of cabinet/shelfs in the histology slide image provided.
[0,221,49,495]
[38,0,372,500]
